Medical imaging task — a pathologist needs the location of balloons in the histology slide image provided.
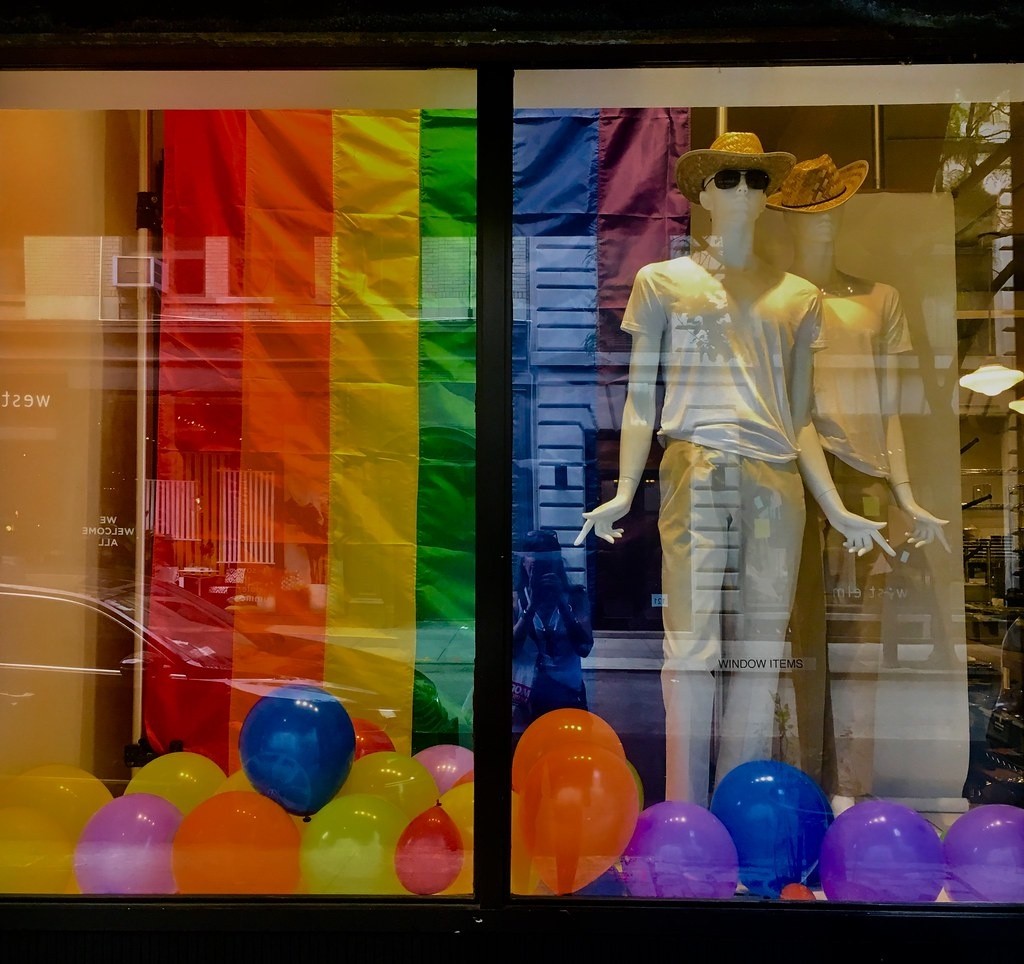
[819,799,944,902]
[942,804,1024,903]
[0,684,835,901]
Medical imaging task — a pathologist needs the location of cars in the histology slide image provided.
[1,564,460,804]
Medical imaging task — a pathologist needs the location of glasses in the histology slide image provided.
[703,169,769,192]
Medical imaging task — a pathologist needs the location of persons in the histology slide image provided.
[572,132,953,809]
[512,530,596,735]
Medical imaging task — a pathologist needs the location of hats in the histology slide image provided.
[765,152,869,214]
[675,131,797,204]
